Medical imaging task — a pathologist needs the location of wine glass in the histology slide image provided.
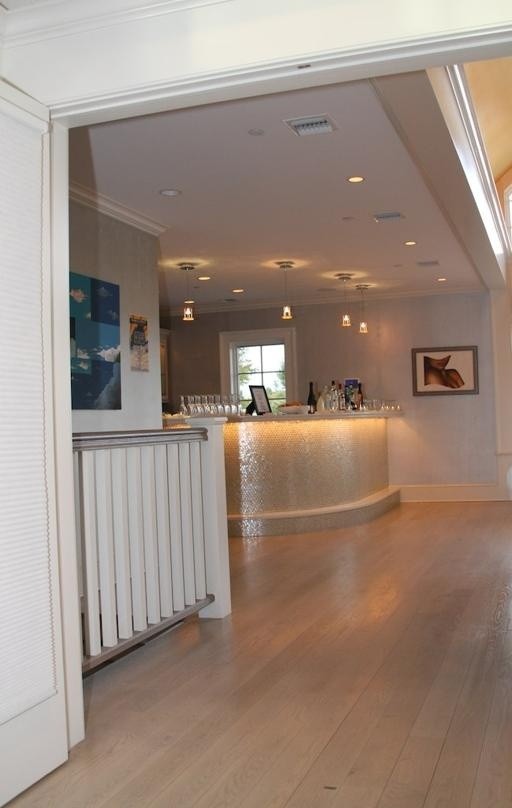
[177,393,243,417]
[363,399,400,413]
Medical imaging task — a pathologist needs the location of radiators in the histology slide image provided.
[77,441,208,659]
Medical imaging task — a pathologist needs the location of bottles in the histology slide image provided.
[331,382,363,411]
[307,381,317,414]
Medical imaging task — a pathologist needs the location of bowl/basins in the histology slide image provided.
[277,404,311,414]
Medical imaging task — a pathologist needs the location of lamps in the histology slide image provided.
[337,276,369,334]
[278,263,294,320]
[177,264,196,322]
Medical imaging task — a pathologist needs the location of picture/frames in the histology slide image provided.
[245,385,273,416]
[411,345,479,396]
